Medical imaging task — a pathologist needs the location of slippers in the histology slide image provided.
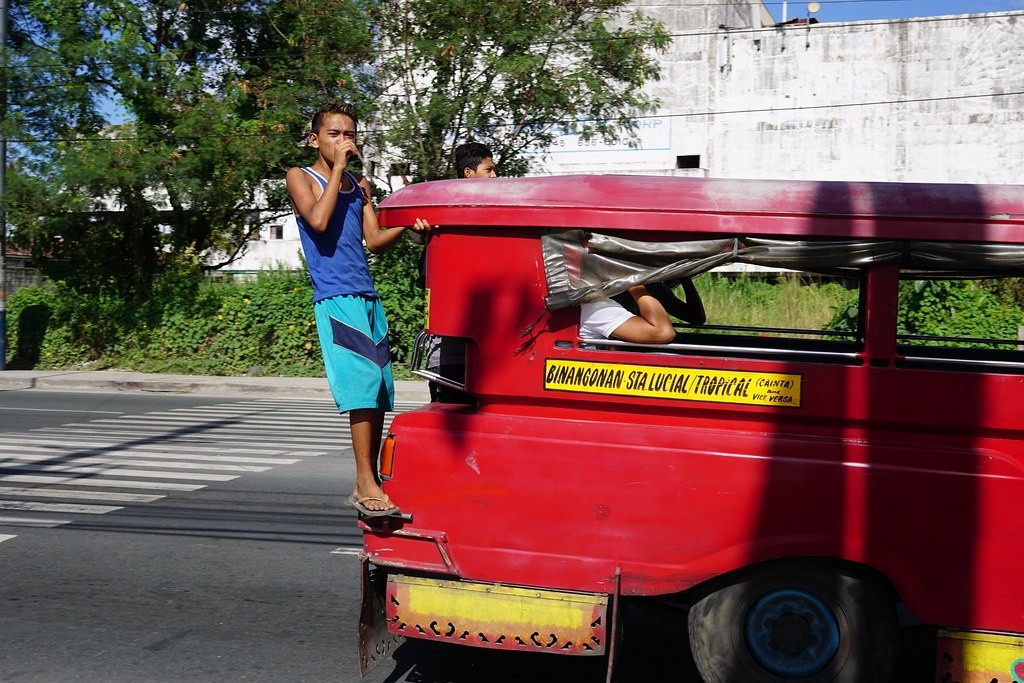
[349,494,399,516]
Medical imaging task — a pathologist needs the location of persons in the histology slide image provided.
[579,262,706,344]
[286,103,439,518]
[455,143,497,178]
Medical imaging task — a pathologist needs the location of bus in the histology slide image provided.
[355,173,1024,682]
